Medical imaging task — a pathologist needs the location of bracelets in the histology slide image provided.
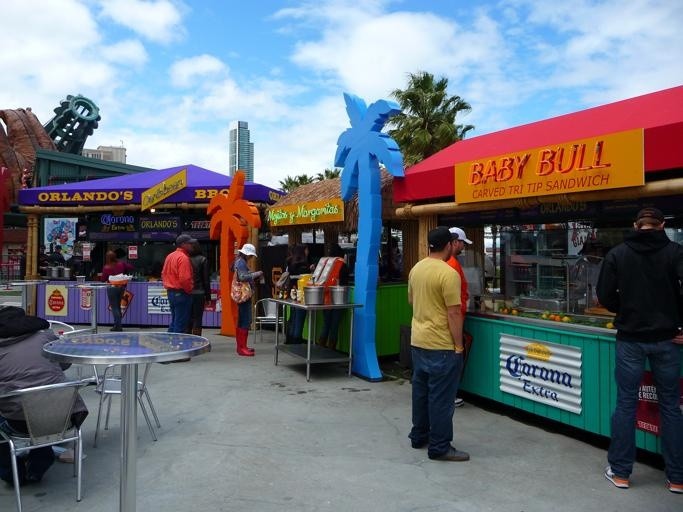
[455,345,464,352]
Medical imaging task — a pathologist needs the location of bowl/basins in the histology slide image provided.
[109,280,128,285]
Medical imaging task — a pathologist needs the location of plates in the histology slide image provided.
[337,243,352,249]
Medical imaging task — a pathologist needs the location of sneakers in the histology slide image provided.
[110,325,123,332]
[605,466,630,487]
[664,473,683,492]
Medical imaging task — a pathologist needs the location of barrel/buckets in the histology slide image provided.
[329,279,350,304]
[46,266,64,277]
[302,285,326,304]
[63,267,74,277]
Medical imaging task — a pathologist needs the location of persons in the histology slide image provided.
[161,234,212,338]
[320,243,350,351]
[563,239,608,308]
[281,241,316,346]
[39,244,65,276]
[446,227,472,408]
[408,227,470,461]
[596,207,683,494]
[382,238,404,282]
[102,251,135,332]
[0,306,88,488]
[484,253,495,288]
[230,244,263,356]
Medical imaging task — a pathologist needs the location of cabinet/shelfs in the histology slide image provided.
[268,295,365,383]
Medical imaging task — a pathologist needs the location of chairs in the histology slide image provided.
[88,358,163,449]
[61,328,100,388]
[252,298,286,344]
[1,374,99,512]
[44,318,72,339]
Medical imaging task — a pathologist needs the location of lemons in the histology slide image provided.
[562,316,570,322]
[607,323,614,329]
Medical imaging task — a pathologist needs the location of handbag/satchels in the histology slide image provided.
[230,269,252,304]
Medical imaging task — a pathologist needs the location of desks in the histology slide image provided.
[5,278,49,317]
[63,283,121,335]
[39,329,214,512]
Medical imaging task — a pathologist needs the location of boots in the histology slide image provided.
[236,326,256,356]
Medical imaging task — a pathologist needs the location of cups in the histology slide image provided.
[46,267,70,278]
[75,275,86,285]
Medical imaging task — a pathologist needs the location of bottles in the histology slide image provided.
[336,232,356,246]
[127,271,144,281]
[277,284,299,299]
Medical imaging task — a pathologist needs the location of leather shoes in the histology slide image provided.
[412,437,428,449]
[428,443,470,462]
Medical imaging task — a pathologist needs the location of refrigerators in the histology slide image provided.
[502,220,594,308]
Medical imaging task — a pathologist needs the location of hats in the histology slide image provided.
[426,226,458,251]
[448,227,473,245]
[238,244,258,258]
[174,233,199,247]
